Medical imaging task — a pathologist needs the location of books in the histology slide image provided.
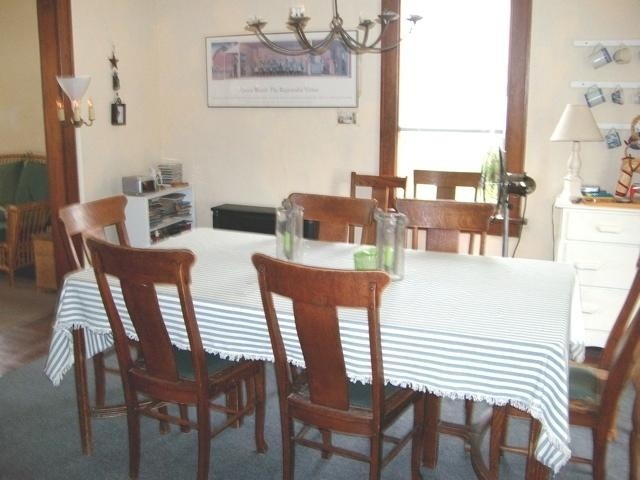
[157,162,182,186]
[149,193,191,228]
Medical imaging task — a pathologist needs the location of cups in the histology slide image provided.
[276,200,303,262]
[374,208,408,281]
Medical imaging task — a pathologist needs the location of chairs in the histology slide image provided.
[349,172,407,242]
[282,194,378,245]
[86,236,268,480]
[251,252,425,479]
[393,198,494,468]
[414,170,486,202]
[489,261,640,480]
[0,202,50,289]
[57,195,170,435]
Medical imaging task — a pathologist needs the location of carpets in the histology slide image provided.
[0,346,640,480]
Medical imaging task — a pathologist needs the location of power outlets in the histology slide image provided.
[337,112,355,124]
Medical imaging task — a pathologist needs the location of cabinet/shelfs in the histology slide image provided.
[211,203,317,240]
[554,201,640,348]
[115,185,195,248]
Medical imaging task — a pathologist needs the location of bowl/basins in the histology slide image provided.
[356,246,395,272]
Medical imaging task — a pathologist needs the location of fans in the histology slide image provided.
[480,146,536,257]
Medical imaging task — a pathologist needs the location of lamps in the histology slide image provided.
[245,0,422,55]
[549,104,604,204]
[55,76,96,127]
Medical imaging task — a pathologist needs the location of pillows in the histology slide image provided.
[0,161,24,209]
[16,161,49,208]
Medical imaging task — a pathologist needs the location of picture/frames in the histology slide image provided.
[206,30,359,108]
